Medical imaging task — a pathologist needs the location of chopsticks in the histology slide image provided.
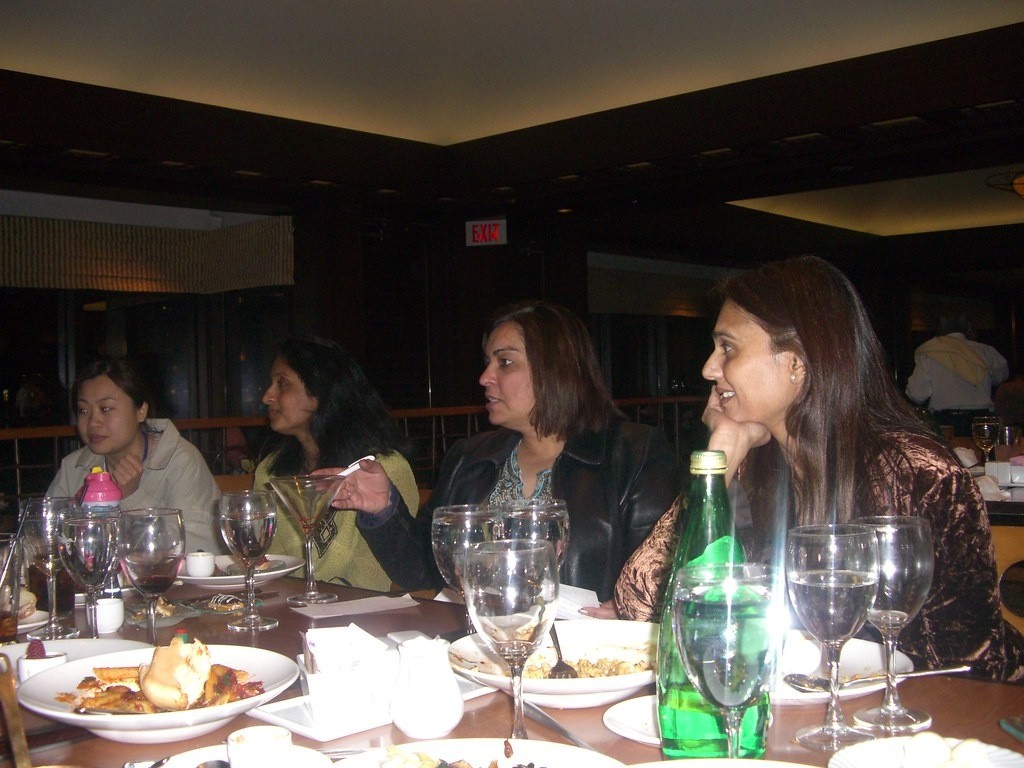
[0,720,101,763]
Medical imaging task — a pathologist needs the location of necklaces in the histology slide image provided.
[105,428,148,484]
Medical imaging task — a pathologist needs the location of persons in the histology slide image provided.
[250,334,420,592]
[617,257,1022,686]
[41,360,229,557]
[306,298,680,622]
[903,299,1009,438]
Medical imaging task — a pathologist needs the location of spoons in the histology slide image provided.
[784,663,971,695]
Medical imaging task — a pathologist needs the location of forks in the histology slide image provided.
[547,618,576,678]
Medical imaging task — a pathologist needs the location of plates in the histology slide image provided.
[738,626,913,705]
[14,606,54,633]
[601,692,773,747]
[0,636,158,694]
[172,548,305,591]
[16,641,301,744]
[330,735,623,768]
[247,669,501,744]
[828,732,1024,766]
[443,620,669,711]
[127,744,333,768]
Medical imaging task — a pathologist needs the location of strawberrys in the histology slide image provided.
[26,640,46,659]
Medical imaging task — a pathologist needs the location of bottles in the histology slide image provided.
[657,447,780,761]
[75,467,122,509]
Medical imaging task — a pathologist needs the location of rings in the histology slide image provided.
[347,489,351,499]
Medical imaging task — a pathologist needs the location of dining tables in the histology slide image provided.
[0,575,476,763]
[0,670,1023,766]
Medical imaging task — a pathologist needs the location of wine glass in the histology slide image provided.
[268,473,348,607]
[216,487,280,633]
[970,416,1001,477]
[785,527,877,753]
[428,494,572,743]
[849,512,932,734]
[16,493,187,645]
[665,556,787,760]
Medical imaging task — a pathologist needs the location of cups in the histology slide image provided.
[187,551,215,577]
[999,425,1021,447]
[1,530,22,643]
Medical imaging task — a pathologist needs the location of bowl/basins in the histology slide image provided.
[214,554,237,573]
